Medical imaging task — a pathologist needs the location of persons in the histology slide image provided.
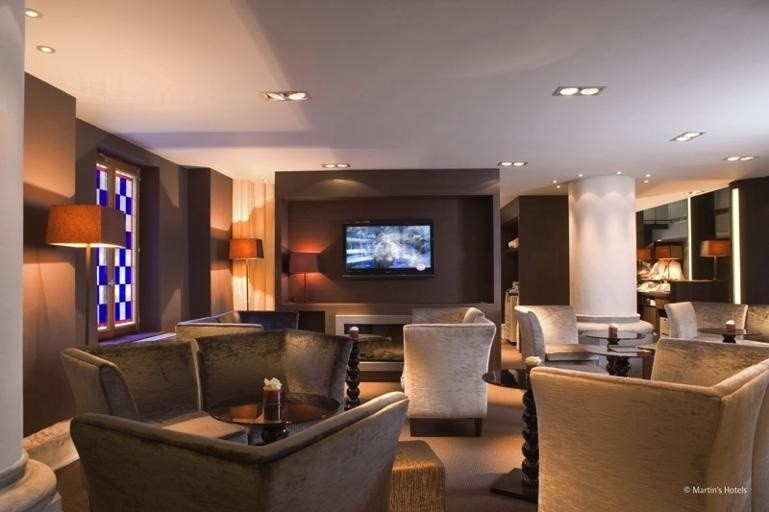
[646,257,685,291]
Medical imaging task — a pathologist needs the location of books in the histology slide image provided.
[606,343,651,353]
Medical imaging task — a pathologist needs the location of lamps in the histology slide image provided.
[699,239,730,281]
[229,239,265,311]
[655,246,683,279]
[45,204,127,346]
[288,252,321,302]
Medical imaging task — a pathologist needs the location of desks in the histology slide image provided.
[482,357,654,504]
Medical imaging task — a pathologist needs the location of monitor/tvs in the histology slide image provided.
[341,218,438,278]
